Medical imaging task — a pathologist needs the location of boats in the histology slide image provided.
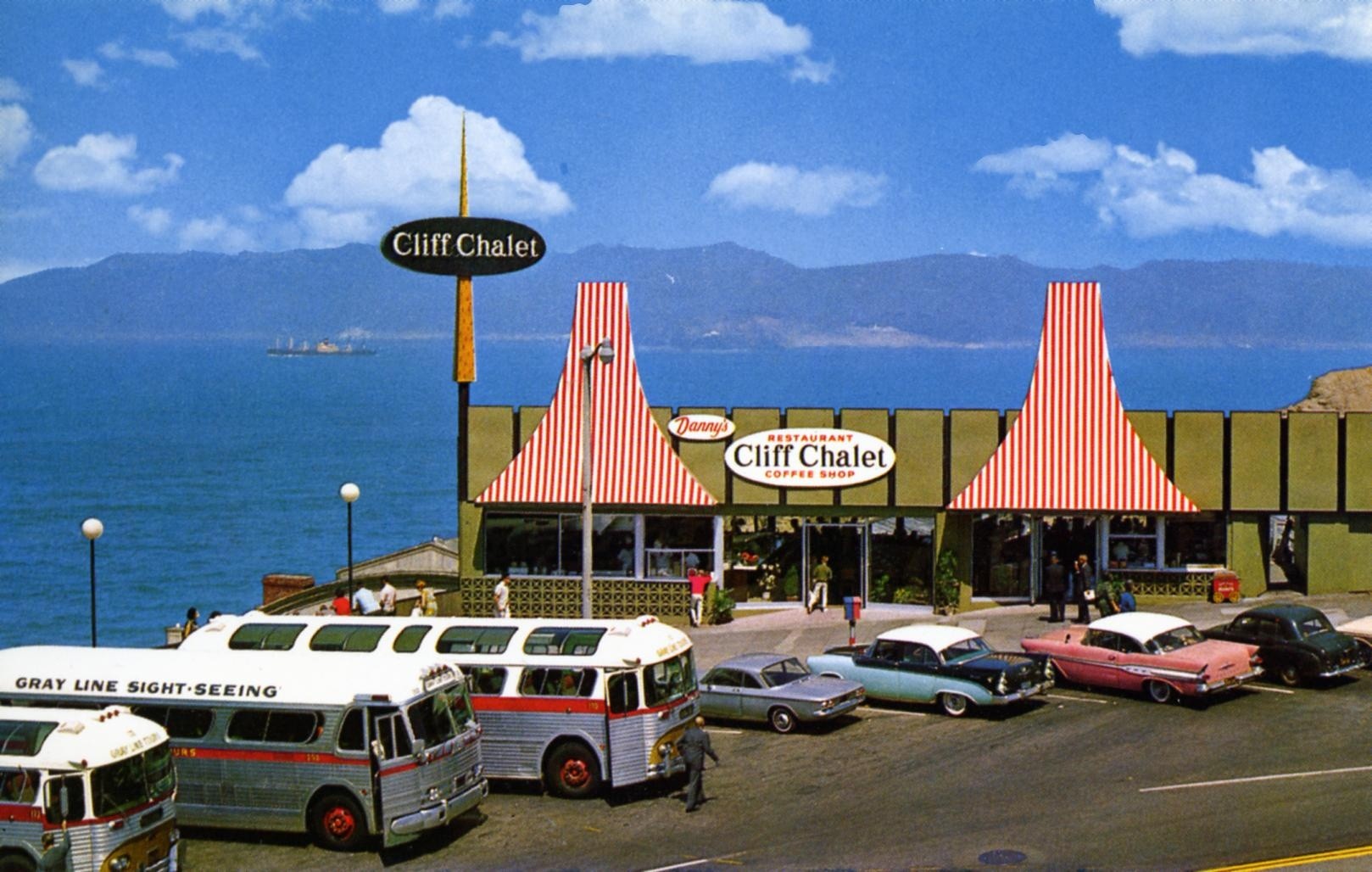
[267,336,376,356]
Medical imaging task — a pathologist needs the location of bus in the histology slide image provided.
[177,609,704,800]
[0,646,488,853]
[0,704,179,872]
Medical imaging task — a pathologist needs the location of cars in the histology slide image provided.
[1197,602,1372,688]
[805,623,1057,718]
[656,652,866,733]
[1020,611,1267,705]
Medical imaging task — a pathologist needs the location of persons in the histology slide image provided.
[319,575,397,616]
[680,551,700,568]
[807,556,833,614]
[1044,551,1092,624]
[617,543,634,577]
[688,569,712,628]
[675,716,720,813]
[491,572,511,618]
[653,537,673,577]
[1098,572,1136,618]
[183,606,222,640]
[410,580,437,617]
[36,818,71,872]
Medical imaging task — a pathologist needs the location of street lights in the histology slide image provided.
[340,482,361,614]
[80,517,104,647]
